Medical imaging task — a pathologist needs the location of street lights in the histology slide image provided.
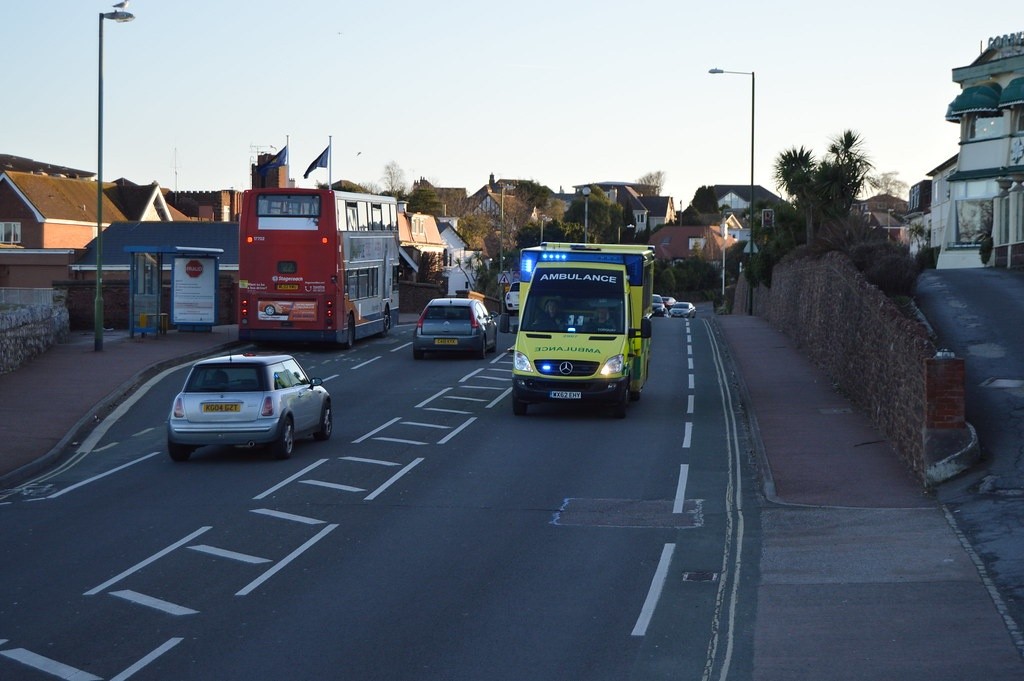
[888,209,894,239]
[618,225,634,245]
[94,12,135,352]
[709,68,755,316]
[540,217,552,243]
[501,186,516,314]
[582,187,591,243]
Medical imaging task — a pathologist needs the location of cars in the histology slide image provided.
[653,294,666,317]
[669,301,696,318]
[165,354,332,461]
[412,298,500,361]
[506,282,520,317]
[662,297,676,312]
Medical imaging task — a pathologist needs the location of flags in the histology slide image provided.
[256,144,287,177]
[303,144,329,179]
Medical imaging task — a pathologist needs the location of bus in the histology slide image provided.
[238,188,407,349]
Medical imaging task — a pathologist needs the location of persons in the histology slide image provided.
[593,307,616,329]
[531,297,564,326]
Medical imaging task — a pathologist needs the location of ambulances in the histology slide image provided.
[512,243,655,419]
